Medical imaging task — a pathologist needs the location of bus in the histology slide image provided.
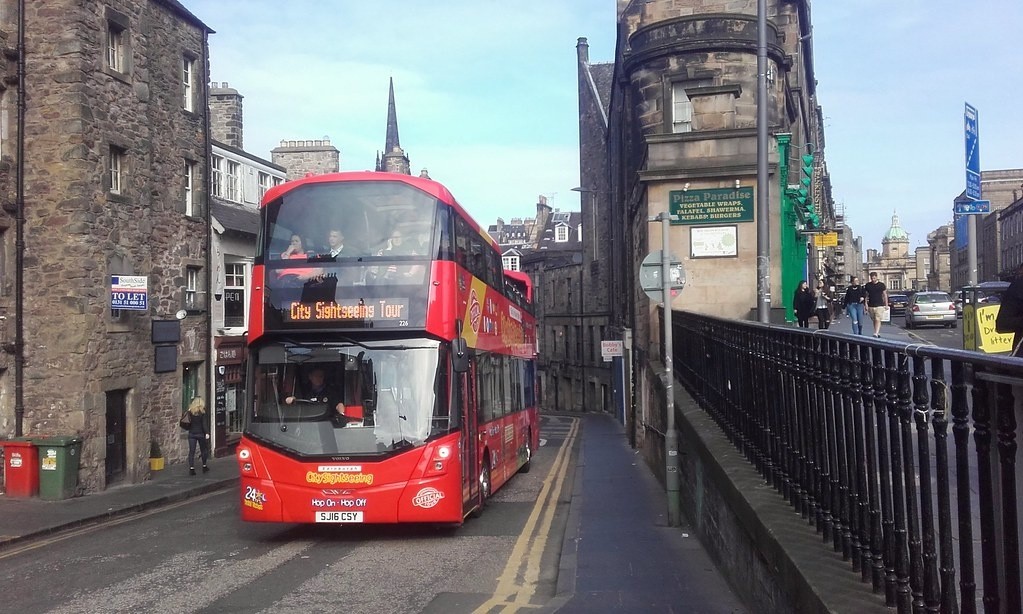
[236,171,539,523]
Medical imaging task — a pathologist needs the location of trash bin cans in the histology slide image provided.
[750,308,787,329]
[0,436,47,498]
[32,435,83,500]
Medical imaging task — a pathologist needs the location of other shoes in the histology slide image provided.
[203,466,209,473]
[190,469,196,475]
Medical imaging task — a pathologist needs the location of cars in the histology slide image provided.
[888,296,910,315]
[954,291,989,315]
[905,291,957,329]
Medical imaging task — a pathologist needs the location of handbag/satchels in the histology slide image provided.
[180,411,191,430]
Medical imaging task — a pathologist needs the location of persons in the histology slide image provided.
[379,229,422,285]
[276,231,361,283]
[281,366,345,416]
[842,272,889,337]
[185,397,209,475]
[996,263,1023,358]
[793,279,831,329]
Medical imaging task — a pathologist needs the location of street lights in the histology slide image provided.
[646,211,681,528]
